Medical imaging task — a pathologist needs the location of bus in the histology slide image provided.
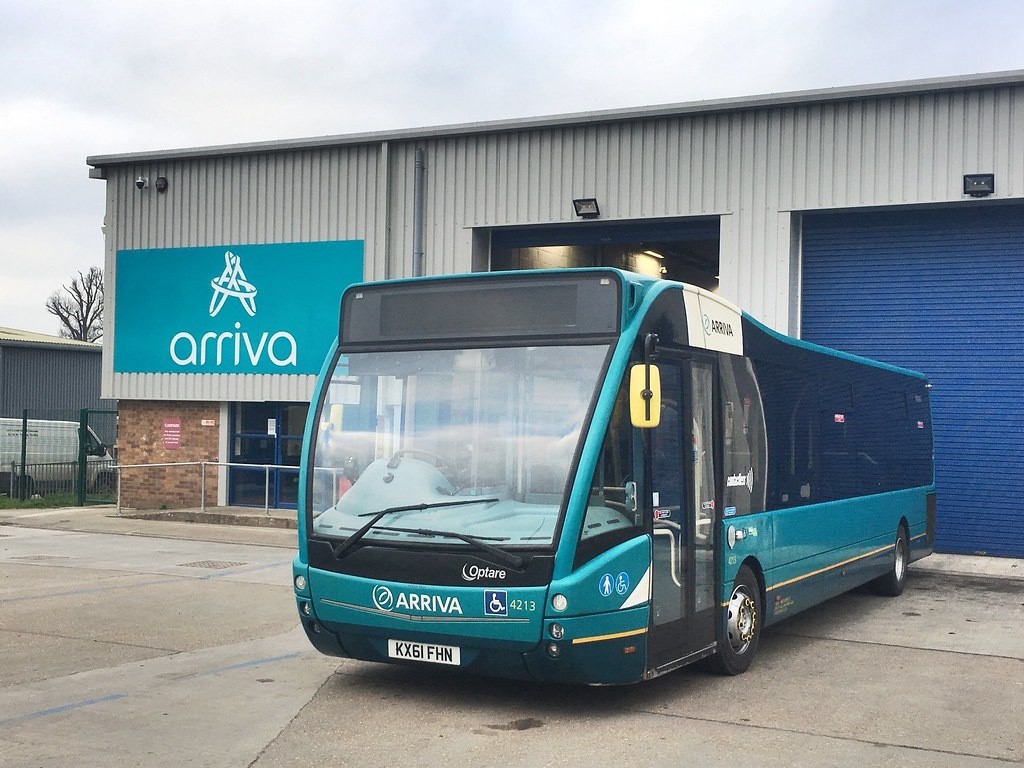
[292,264,937,695]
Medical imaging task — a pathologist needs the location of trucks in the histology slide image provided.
[0,418,116,499]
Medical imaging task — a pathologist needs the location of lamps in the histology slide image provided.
[573,197,600,220]
[962,173,994,198]
